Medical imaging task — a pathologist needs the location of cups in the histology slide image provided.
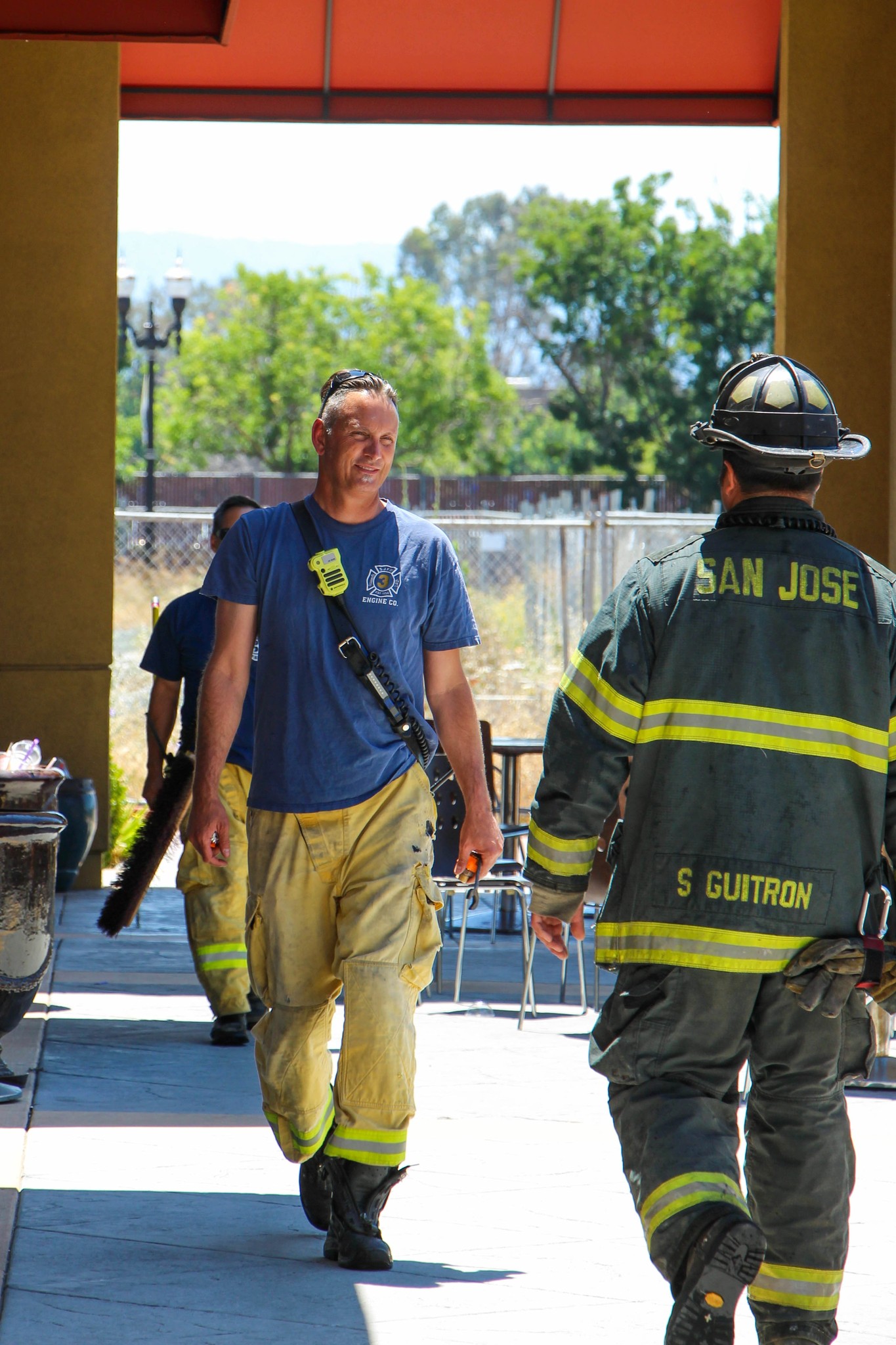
[0,752,11,771]
[10,739,42,771]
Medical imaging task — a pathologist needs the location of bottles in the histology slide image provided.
[455,851,480,884]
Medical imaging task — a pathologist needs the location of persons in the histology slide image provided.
[187,369,504,1271]
[521,356,896,1345]
[139,496,269,1047]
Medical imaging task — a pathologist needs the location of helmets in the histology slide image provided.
[689,355,870,458]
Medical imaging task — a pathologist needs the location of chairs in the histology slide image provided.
[424,752,623,1027]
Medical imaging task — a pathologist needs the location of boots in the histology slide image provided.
[210,1013,250,1044]
[299,1083,331,1231]
[665,1204,767,1345]
[245,986,268,1022]
[324,1156,409,1270]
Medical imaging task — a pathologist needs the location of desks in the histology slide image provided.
[436,735,547,938]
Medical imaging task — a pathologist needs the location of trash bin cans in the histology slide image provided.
[0,764,65,811]
[56,778,98,894]
[0,811,68,1088]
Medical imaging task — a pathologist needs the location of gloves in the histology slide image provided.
[783,937,896,1018]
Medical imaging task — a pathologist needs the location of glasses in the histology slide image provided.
[214,528,230,539]
[318,370,384,419]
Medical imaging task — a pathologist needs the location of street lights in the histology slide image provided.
[117,254,192,569]
